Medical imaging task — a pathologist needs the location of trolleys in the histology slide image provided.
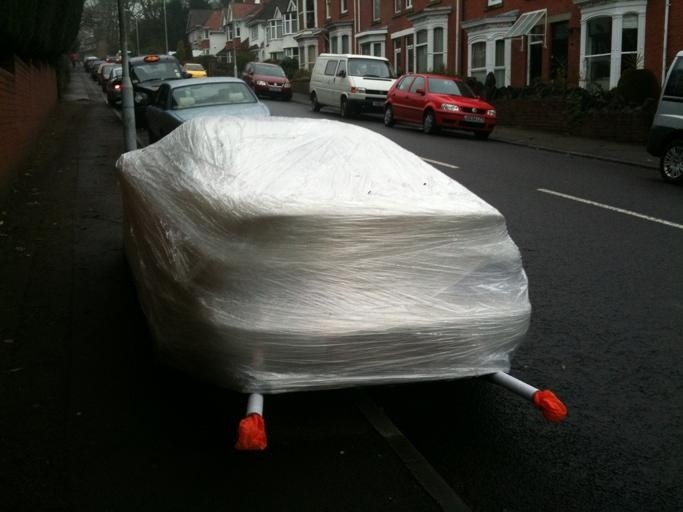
[99,110,568,447]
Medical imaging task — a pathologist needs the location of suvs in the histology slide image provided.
[644,49,682,182]
